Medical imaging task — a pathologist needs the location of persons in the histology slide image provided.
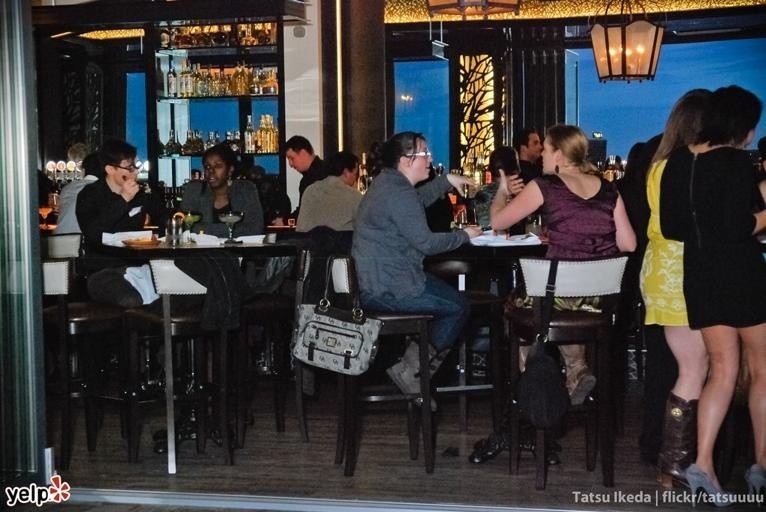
[32,120,678,466]
[639,88,713,488]
[660,85,766,511]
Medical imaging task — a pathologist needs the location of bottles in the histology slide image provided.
[461,151,492,188]
[165,113,278,155]
[163,185,181,207]
[358,165,369,193]
[597,155,626,182]
[166,55,278,98]
[166,23,274,50]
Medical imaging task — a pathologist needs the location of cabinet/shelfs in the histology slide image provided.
[146,17,284,212]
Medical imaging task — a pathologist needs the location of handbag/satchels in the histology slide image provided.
[514,335,571,432]
[291,303,384,378]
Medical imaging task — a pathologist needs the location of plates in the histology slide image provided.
[45,159,83,173]
[135,160,148,172]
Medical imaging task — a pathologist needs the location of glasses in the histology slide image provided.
[399,148,432,161]
[104,160,142,173]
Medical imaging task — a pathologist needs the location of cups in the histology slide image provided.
[288,219,295,227]
[164,216,181,247]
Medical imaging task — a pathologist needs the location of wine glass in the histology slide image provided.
[218,209,244,246]
[179,210,204,247]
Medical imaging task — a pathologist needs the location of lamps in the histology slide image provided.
[587,0,663,83]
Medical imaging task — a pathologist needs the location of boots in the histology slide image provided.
[387,341,450,413]
[655,389,698,489]
[557,344,596,407]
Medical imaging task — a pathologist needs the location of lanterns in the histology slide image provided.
[589,18,666,83]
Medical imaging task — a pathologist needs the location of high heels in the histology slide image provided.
[684,464,737,508]
[745,464,765,506]
